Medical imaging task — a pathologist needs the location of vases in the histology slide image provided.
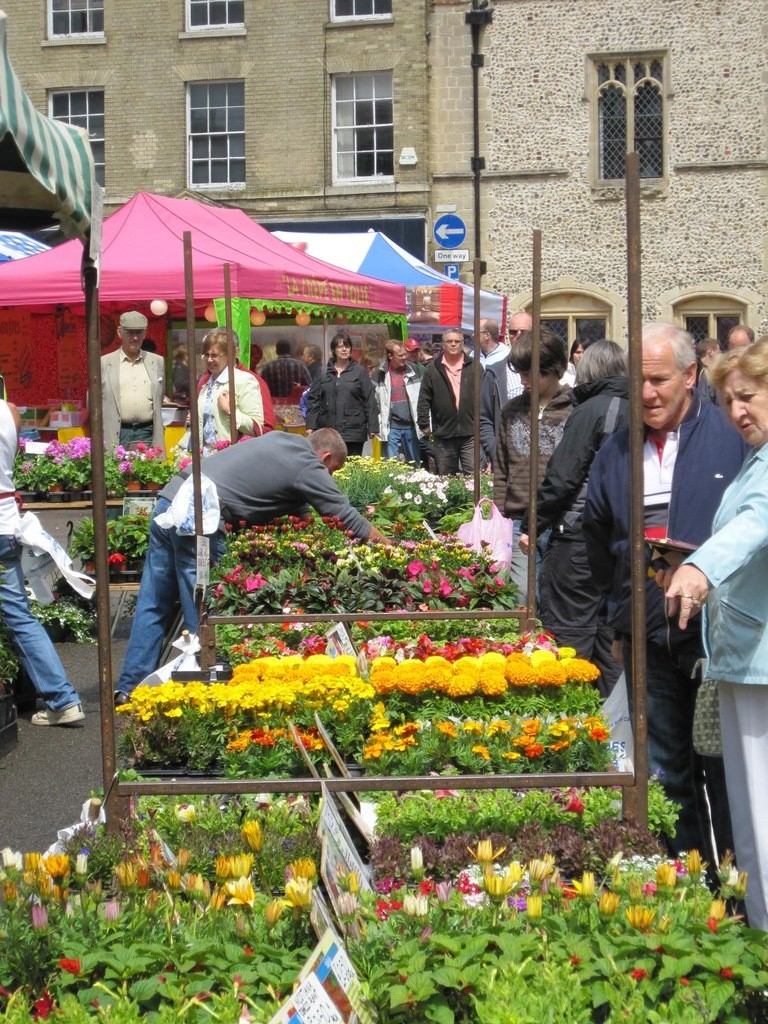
[49,483,63,503]
[127,482,142,497]
[68,483,82,501]
[22,490,36,503]
[146,481,163,497]
[110,562,128,583]
[88,483,94,501]
[85,561,96,571]
[131,560,143,582]
[42,617,69,642]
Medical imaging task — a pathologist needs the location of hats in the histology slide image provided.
[404,338,420,351]
[120,311,148,331]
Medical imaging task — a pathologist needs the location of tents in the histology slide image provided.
[167,229,506,379]
[0,230,53,264]
[0,9,118,794]
[0,194,410,414]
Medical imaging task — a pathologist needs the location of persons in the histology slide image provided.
[0,378,86,725]
[85,311,168,462]
[582,322,750,927]
[114,427,393,707]
[138,311,768,782]
[665,338,768,942]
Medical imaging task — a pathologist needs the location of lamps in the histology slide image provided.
[150,299,168,316]
[250,307,265,327]
[204,301,217,323]
[295,311,311,327]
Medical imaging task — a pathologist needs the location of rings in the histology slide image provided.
[693,597,700,601]
[700,595,707,603]
[681,596,693,600]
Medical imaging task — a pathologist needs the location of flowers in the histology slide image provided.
[0,437,768,1024]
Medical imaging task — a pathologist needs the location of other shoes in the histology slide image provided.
[113,691,129,705]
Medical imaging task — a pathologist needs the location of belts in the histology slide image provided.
[122,421,154,429]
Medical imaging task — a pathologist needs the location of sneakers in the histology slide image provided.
[30,703,85,726]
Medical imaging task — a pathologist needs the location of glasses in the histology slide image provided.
[443,339,462,344]
[509,329,526,336]
[203,353,226,359]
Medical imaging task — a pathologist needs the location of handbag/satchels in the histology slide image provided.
[458,497,513,581]
[690,656,722,757]
[596,670,635,776]
[300,366,327,420]
